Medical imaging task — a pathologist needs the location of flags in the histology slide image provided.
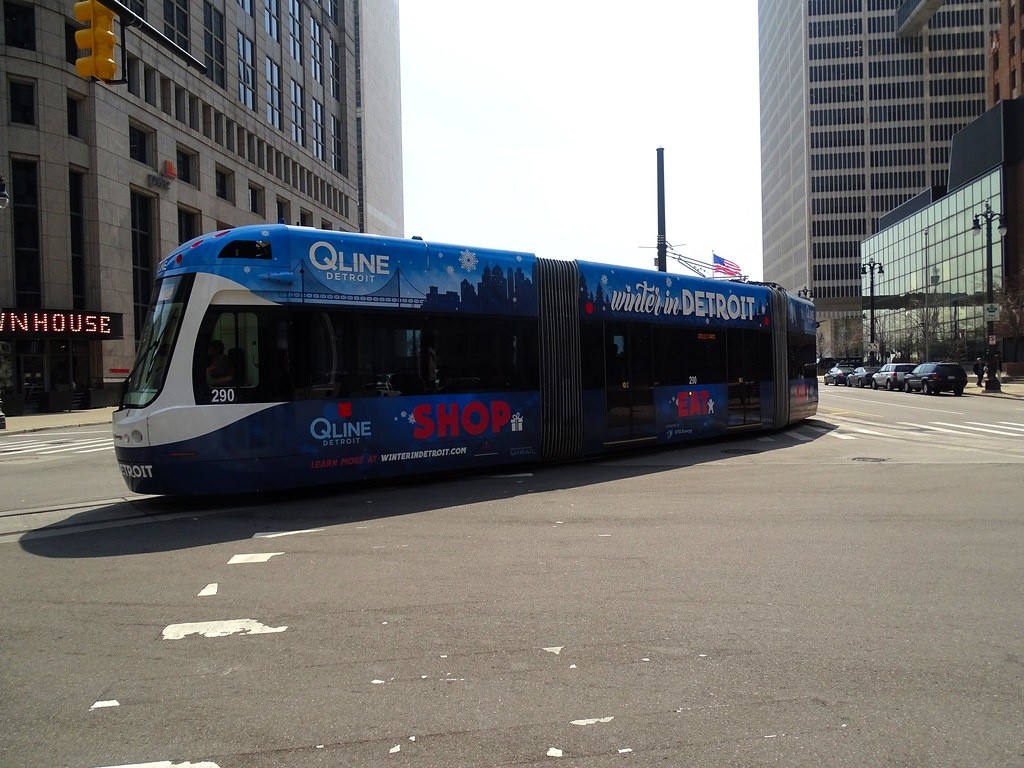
[713,254,741,276]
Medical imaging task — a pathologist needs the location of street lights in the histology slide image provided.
[971,199,1007,393]
[859,257,884,367]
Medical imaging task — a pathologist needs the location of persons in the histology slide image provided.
[993,351,1003,386]
[973,358,985,387]
[206,339,246,387]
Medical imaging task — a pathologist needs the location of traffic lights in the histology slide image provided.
[74,0,117,87]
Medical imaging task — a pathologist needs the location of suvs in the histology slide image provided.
[903,362,968,396]
[870,364,918,391]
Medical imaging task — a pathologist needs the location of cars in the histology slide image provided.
[846,367,880,388]
[824,367,854,386]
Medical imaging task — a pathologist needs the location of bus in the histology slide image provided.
[113,224,818,500]
